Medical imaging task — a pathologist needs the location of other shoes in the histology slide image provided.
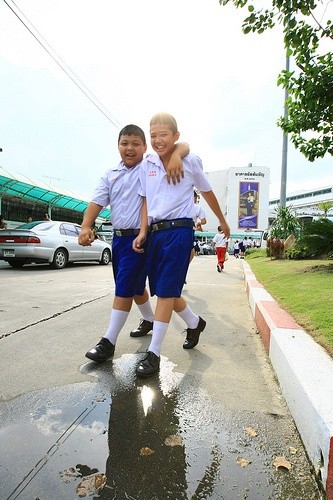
[216,264,222,272]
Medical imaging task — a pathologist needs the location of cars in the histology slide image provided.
[0,221,112,270]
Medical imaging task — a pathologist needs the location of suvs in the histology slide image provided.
[95,222,114,245]
[0,220,26,230]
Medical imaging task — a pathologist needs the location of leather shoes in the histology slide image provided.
[85,337,115,361]
[130,319,154,337]
[181,316,207,349]
[136,351,161,377]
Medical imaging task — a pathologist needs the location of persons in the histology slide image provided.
[77,124,190,363]
[0,215,7,230]
[212,225,230,272]
[234,237,261,259]
[193,239,217,256]
[183,190,207,284]
[43,213,52,221]
[26,216,33,223]
[133,112,229,378]
[245,192,255,215]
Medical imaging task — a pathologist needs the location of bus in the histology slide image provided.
[194,231,264,255]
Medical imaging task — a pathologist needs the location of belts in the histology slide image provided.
[150,219,195,230]
[113,228,139,236]
